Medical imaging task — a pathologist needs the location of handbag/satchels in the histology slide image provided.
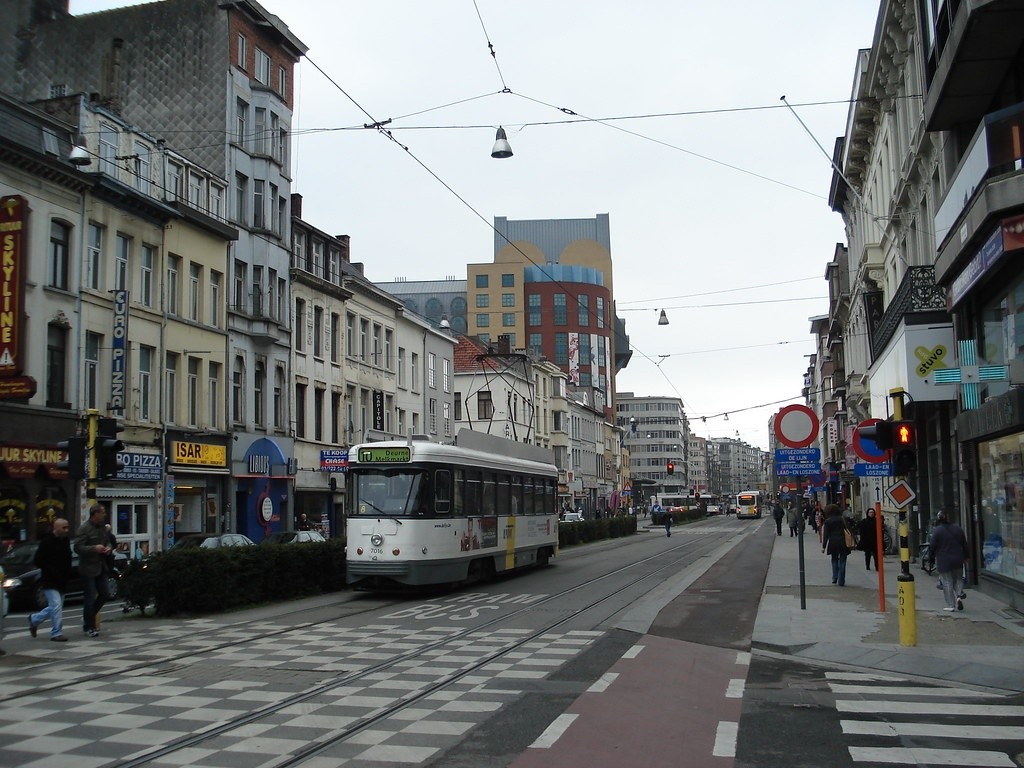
[841,516,855,547]
[670,518,673,523]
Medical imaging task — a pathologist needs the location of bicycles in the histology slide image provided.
[918,528,938,576]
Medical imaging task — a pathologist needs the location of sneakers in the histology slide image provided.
[85,629,99,637]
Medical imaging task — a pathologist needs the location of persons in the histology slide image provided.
[929,511,968,611]
[298,514,314,531]
[74,504,112,637]
[28,518,72,642]
[559,506,652,521]
[801,502,852,543]
[821,504,851,586]
[859,508,879,571]
[787,504,797,537]
[0,566,9,656]
[664,508,672,537]
[726,507,730,516]
[461,518,480,550]
[773,502,784,536]
[105,523,149,570]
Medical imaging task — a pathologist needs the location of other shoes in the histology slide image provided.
[28,613,37,637]
[831,579,837,583]
[50,635,67,642]
[956,597,963,611]
[943,607,955,612]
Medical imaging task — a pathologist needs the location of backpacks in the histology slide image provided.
[654,506,659,512]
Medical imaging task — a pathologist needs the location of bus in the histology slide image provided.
[728,495,737,513]
[736,491,762,519]
[650,494,720,516]
[344,441,561,591]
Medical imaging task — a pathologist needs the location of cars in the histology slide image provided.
[0,540,130,611]
[0,566,9,621]
[139,533,258,569]
[257,531,326,544]
[558,513,585,523]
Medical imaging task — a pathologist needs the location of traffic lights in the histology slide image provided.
[631,425,636,433]
[776,491,781,499]
[97,417,126,477]
[57,437,85,479]
[667,464,674,475]
[95,436,124,480]
[892,419,919,476]
[859,421,889,450]
[695,493,700,502]
